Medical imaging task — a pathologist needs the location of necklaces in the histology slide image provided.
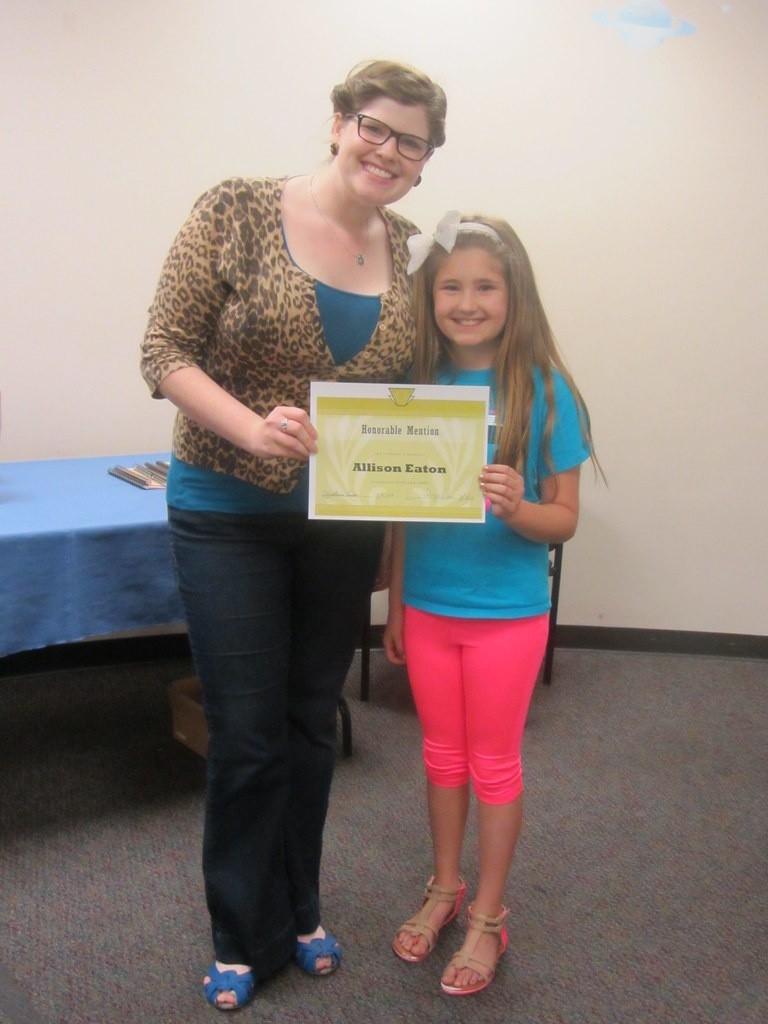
[310,172,370,265]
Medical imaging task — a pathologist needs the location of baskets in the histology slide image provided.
[171,678,208,760]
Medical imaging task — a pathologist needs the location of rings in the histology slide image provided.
[280,418,289,432]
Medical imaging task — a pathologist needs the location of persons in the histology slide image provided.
[381,210,592,998]
[137,58,448,1011]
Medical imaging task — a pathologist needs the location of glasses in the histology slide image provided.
[348,113,435,161]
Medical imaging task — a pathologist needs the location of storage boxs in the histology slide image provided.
[168,676,210,759]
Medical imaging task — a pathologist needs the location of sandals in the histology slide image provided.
[393,874,466,961]
[288,926,342,976]
[441,900,507,995]
[203,960,258,1011]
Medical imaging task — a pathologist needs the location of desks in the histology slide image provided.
[0,453,354,759]
